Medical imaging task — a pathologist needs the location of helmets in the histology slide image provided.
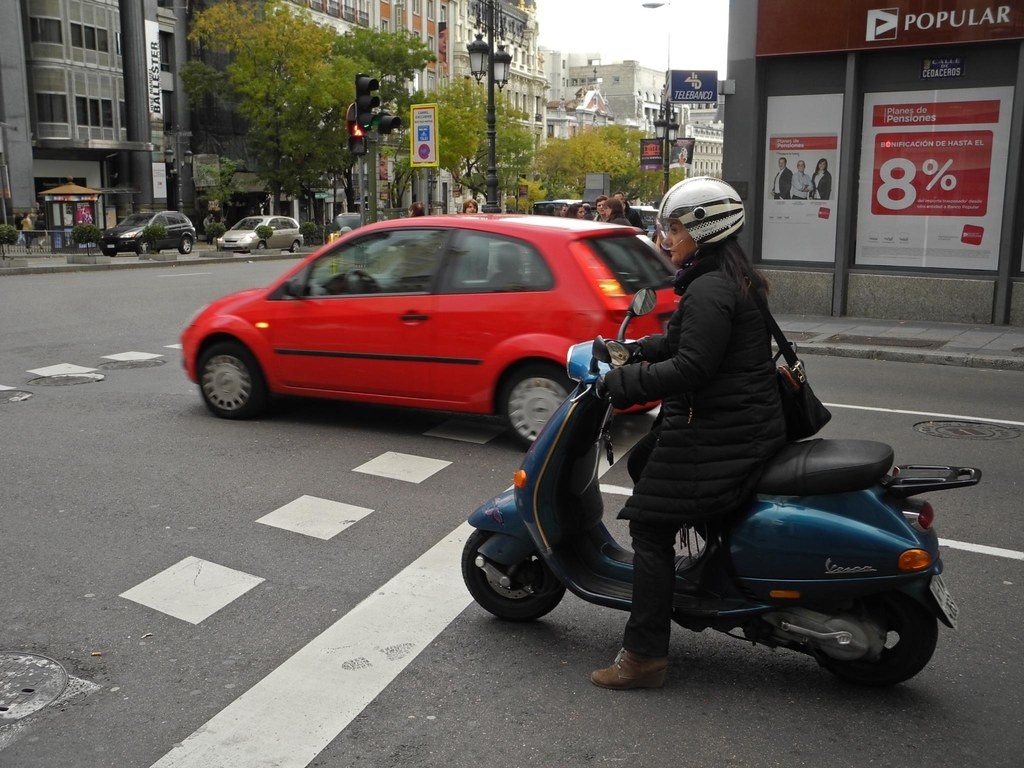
[657,177,745,260]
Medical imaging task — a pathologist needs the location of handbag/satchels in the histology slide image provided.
[774,358,832,443]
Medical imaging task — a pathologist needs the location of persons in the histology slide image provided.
[772,157,832,200]
[407,200,425,217]
[203,213,215,245]
[15,201,47,254]
[463,198,478,213]
[544,192,644,229]
[380,154,388,179]
[590,175,789,690]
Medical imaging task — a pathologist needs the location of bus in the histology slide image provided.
[533,200,658,233]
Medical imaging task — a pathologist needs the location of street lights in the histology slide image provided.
[466,33,511,212]
[652,100,679,193]
[164,143,193,213]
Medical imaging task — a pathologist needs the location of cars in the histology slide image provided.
[179,215,684,452]
[216,216,304,252]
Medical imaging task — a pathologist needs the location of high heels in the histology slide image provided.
[591,652,669,689]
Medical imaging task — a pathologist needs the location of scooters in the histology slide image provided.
[460,285,981,689]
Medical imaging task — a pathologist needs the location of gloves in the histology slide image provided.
[636,336,649,359]
[595,375,609,400]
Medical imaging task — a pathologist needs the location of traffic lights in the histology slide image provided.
[355,74,380,128]
[348,121,367,155]
[378,113,402,135]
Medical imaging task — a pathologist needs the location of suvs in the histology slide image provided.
[99,210,197,257]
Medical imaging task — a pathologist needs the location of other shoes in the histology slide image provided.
[38,243,43,249]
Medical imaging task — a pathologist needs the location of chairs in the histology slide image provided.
[440,242,527,289]
